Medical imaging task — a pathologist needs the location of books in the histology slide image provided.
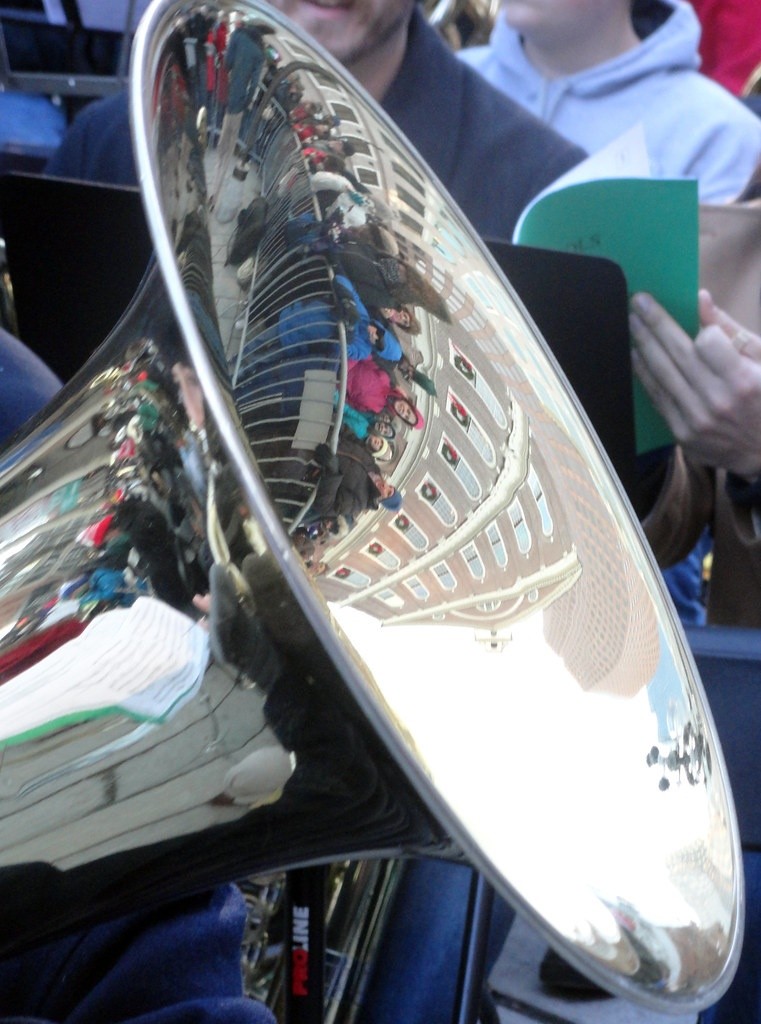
[509,121,700,454]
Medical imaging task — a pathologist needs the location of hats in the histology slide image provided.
[380,485,401,510]
[326,513,348,539]
[397,305,421,335]
[371,436,393,462]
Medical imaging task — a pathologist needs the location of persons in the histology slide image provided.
[38,0,587,245]
[450,0,761,206]
[59,6,473,606]
[628,285,761,631]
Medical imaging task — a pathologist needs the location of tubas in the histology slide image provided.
[0,0,748,1012]
